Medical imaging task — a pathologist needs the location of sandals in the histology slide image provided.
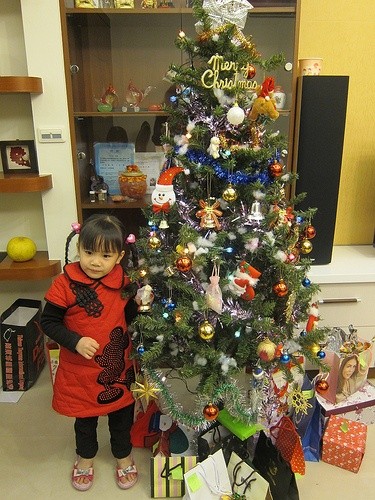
[72,456,94,490]
[115,455,138,490]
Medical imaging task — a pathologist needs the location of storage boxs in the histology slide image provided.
[316,381,375,425]
[218,410,257,440]
[321,415,368,473]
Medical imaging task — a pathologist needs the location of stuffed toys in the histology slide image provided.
[249,78,280,123]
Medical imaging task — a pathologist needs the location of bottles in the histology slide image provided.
[273,85,286,109]
[120,164,147,199]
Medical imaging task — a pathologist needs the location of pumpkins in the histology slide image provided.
[7,237,37,262]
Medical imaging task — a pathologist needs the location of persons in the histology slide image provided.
[334,355,368,402]
[40,214,143,490]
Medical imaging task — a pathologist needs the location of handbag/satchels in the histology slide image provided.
[227,450,273,500]
[212,434,249,467]
[0,299,47,391]
[251,430,299,500]
[198,420,232,462]
[46,336,60,387]
[269,416,305,475]
[315,336,373,404]
[150,440,201,498]
[182,448,232,500]
[292,372,329,463]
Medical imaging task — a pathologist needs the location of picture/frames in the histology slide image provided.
[0,139,39,174]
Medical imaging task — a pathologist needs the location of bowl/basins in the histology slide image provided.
[299,58,323,76]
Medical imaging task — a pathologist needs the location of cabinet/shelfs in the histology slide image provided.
[0,75,63,280]
[294,245,375,379]
[60,0,301,226]
